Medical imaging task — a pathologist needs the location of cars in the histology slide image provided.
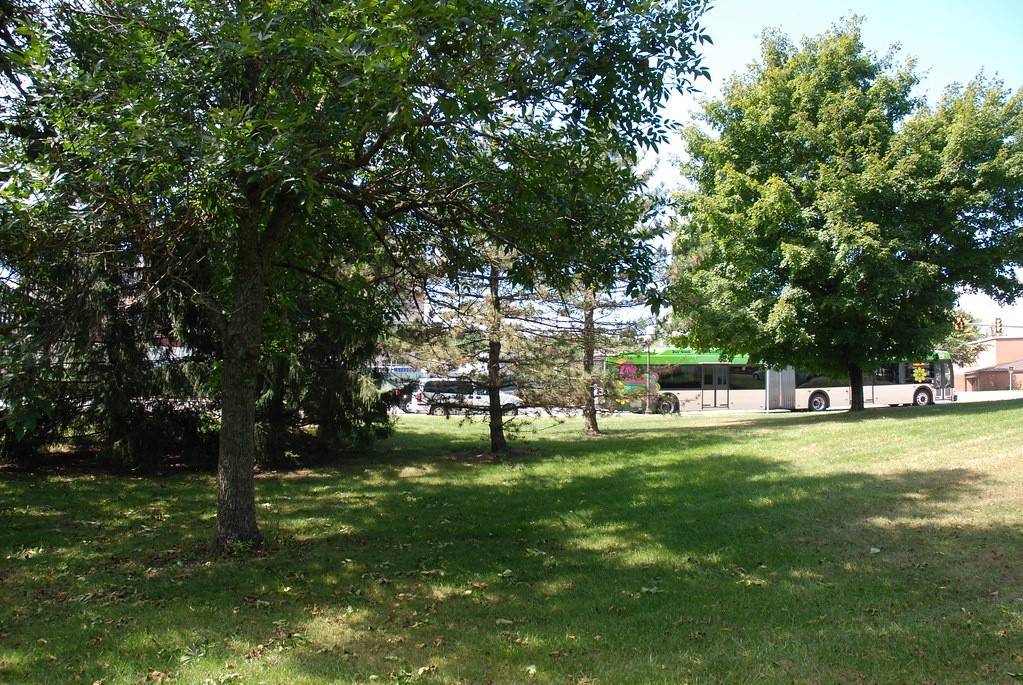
[392,383,419,415]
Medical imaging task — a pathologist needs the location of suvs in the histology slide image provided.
[415,376,526,417]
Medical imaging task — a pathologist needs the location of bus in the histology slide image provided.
[602,347,963,415]
